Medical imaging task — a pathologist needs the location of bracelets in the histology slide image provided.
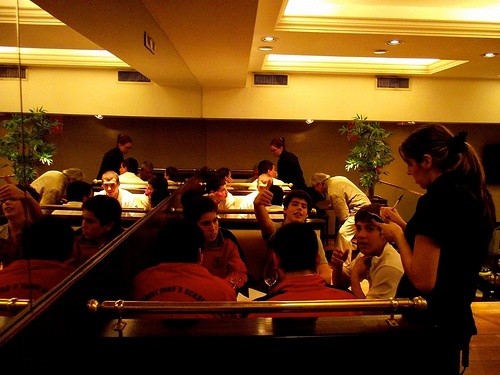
[23,191,30,199]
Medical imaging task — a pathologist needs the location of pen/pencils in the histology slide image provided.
[391,193,404,211]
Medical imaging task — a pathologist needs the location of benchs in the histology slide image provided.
[0,170,430,375]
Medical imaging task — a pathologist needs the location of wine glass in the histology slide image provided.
[264,265,279,292]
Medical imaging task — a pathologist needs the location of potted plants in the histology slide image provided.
[338,114,396,215]
[0,106,64,191]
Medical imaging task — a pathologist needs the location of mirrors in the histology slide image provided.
[0,0,204,337]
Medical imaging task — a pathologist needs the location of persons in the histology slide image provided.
[0,133,404,318]
[372,123,496,375]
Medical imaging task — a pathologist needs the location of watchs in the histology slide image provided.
[59,200,64,204]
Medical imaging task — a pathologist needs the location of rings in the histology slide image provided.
[380,210,382,214]
